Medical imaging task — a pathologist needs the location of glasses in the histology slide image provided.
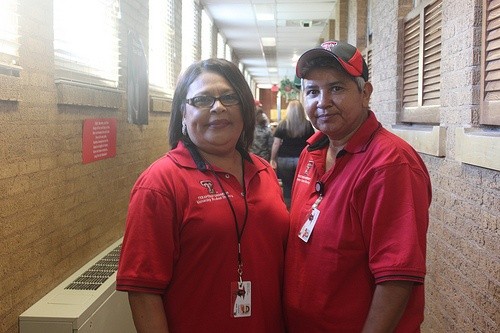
[182,94,242,108]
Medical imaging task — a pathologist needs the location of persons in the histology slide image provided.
[268,100,315,212]
[114,59,291,333]
[249,109,275,162]
[282,40,432,333]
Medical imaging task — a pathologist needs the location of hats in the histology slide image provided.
[295,39,370,82]
